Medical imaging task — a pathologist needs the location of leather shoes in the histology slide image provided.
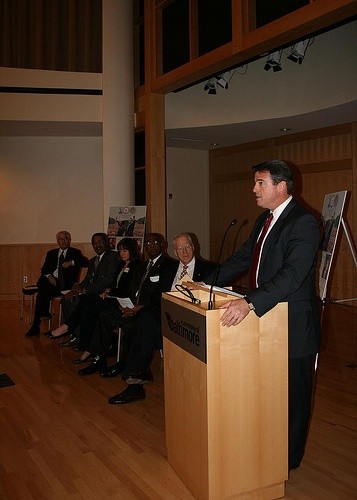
[122,366,153,385]
[78,355,108,376]
[73,345,80,351]
[108,387,146,405]
[100,362,122,377]
[59,335,79,347]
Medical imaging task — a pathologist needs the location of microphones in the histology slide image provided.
[208,219,238,309]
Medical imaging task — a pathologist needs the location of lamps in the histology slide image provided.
[203,74,229,96]
[216,69,235,90]
[286,38,310,65]
[263,50,283,72]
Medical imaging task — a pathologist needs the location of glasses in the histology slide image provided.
[175,244,192,250]
[57,237,71,241]
[144,240,161,246]
[93,240,106,244]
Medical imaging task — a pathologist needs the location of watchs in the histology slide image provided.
[244,297,254,310]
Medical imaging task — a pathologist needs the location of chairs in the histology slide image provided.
[48,290,71,330]
[20,285,39,326]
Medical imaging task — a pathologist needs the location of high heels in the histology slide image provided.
[44,331,67,339]
[72,354,92,364]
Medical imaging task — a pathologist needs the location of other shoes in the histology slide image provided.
[288,455,302,471]
[25,327,41,336]
[40,313,53,321]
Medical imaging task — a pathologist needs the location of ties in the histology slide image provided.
[250,213,273,291]
[90,255,100,285]
[58,251,65,279]
[136,261,153,304]
[179,265,188,280]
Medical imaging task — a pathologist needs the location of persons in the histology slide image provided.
[185,161,321,473]
[116,215,136,236]
[26,230,218,404]
